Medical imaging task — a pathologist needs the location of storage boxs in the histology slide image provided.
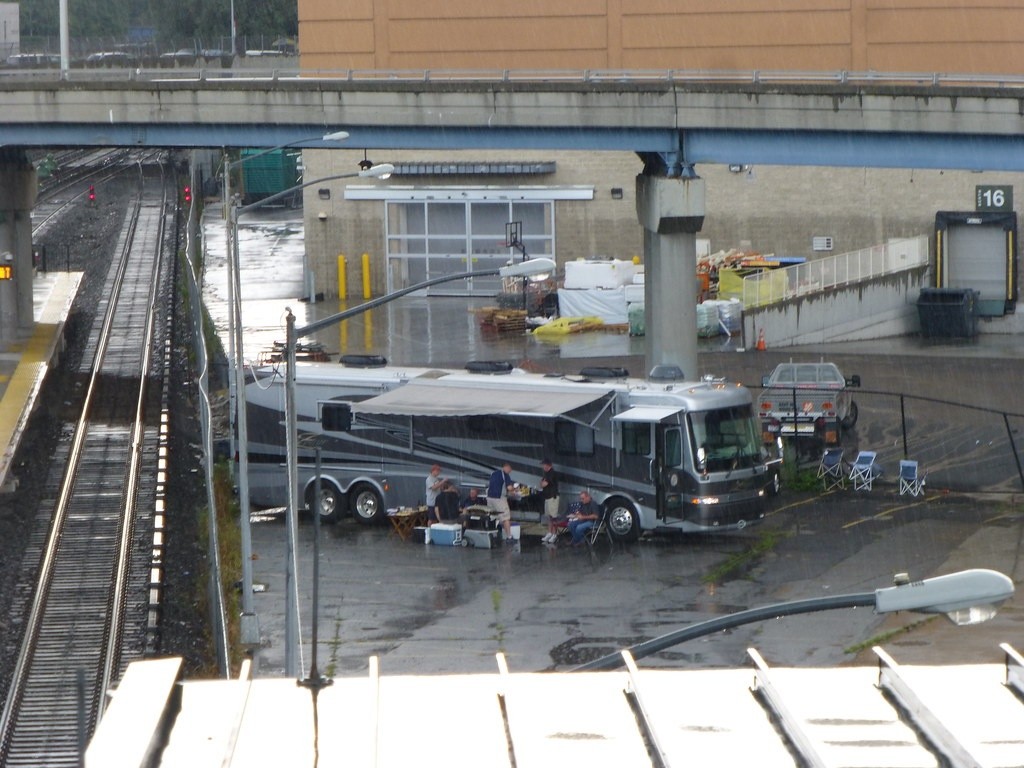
[430,523,463,546]
[464,529,498,549]
[502,522,520,540]
[563,260,645,311]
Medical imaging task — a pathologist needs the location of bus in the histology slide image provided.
[235,352,770,543]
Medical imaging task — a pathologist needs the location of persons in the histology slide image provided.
[539,458,561,542]
[567,491,601,547]
[425,463,520,544]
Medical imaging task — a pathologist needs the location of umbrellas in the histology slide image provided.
[272,36,297,46]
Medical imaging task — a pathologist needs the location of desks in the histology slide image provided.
[387,510,429,542]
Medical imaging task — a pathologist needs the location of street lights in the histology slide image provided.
[231,162,395,393]
[224,130,350,360]
[573,568,1014,672]
[286,257,560,675]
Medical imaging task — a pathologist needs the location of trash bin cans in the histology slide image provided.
[916,287,981,339]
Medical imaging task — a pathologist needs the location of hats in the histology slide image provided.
[540,458,551,465]
[432,465,442,471]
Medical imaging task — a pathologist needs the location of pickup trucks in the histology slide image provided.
[756,362,862,447]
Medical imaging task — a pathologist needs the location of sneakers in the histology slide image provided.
[506,535,518,544]
[541,532,559,544]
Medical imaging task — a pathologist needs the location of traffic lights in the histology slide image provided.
[185,186,191,202]
[89,184,94,201]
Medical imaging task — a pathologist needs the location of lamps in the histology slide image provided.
[318,189,330,199]
[729,164,744,172]
[611,189,622,199]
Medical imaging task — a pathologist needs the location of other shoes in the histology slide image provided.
[565,540,585,547]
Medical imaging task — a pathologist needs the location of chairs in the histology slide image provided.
[897,460,929,497]
[816,448,852,492]
[849,451,883,491]
[551,502,613,546]
